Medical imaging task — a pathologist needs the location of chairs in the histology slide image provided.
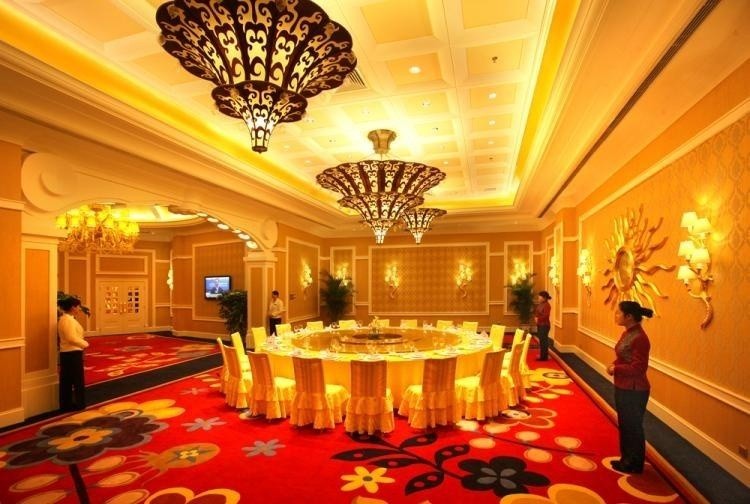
[307,321,323,329]
[251,326,268,350]
[436,319,454,328]
[462,321,478,333]
[222,345,252,409]
[397,356,457,429]
[400,319,418,327]
[499,343,524,407]
[502,334,530,402]
[231,331,248,362]
[373,319,390,326]
[490,324,505,347]
[457,350,504,421]
[247,350,296,420]
[338,320,357,328]
[504,328,524,359]
[216,338,250,394]
[289,357,349,429]
[275,323,292,335]
[344,360,395,433]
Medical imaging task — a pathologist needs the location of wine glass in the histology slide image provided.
[294,320,446,357]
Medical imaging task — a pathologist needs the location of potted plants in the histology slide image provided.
[317,267,358,326]
[504,270,538,341]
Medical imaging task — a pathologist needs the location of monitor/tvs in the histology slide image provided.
[204,275,231,300]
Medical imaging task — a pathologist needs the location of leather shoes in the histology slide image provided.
[611,460,642,472]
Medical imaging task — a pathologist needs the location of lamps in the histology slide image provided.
[575,248,593,299]
[673,211,716,329]
[55,202,141,254]
[449,258,474,298]
[156,0,357,153]
[548,255,560,294]
[299,260,313,301]
[317,129,446,245]
[399,207,447,245]
[384,263,400,299]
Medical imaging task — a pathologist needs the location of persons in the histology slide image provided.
[211,279,224,294]
[534,291,551,360]
[606,301,653,473]
[268,291,284,336]
[57,297,89,413]
